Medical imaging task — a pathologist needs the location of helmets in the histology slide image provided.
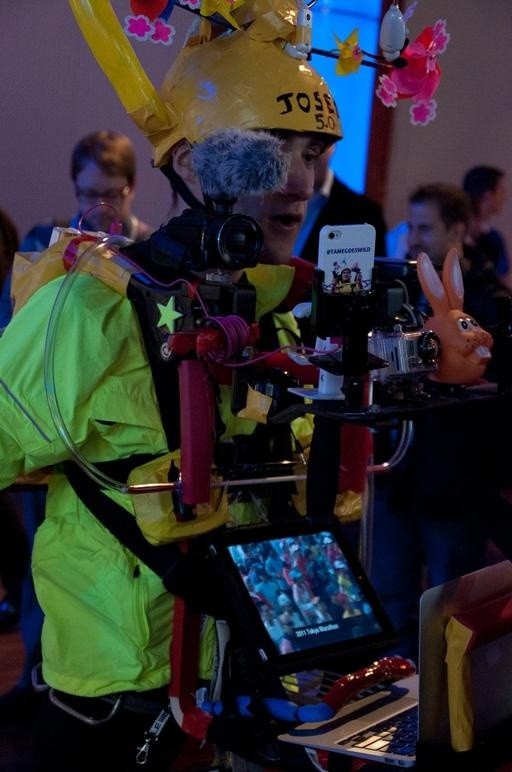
[152,31,342,165]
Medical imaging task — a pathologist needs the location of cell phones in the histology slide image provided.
[319,223,377,292]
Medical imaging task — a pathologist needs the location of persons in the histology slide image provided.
[1,0,511,772]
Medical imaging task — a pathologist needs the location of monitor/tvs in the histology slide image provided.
[218,517,399,676]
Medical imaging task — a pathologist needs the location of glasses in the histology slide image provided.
[75,181,131,205]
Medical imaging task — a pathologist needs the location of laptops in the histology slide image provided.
[275,560,512,768]
